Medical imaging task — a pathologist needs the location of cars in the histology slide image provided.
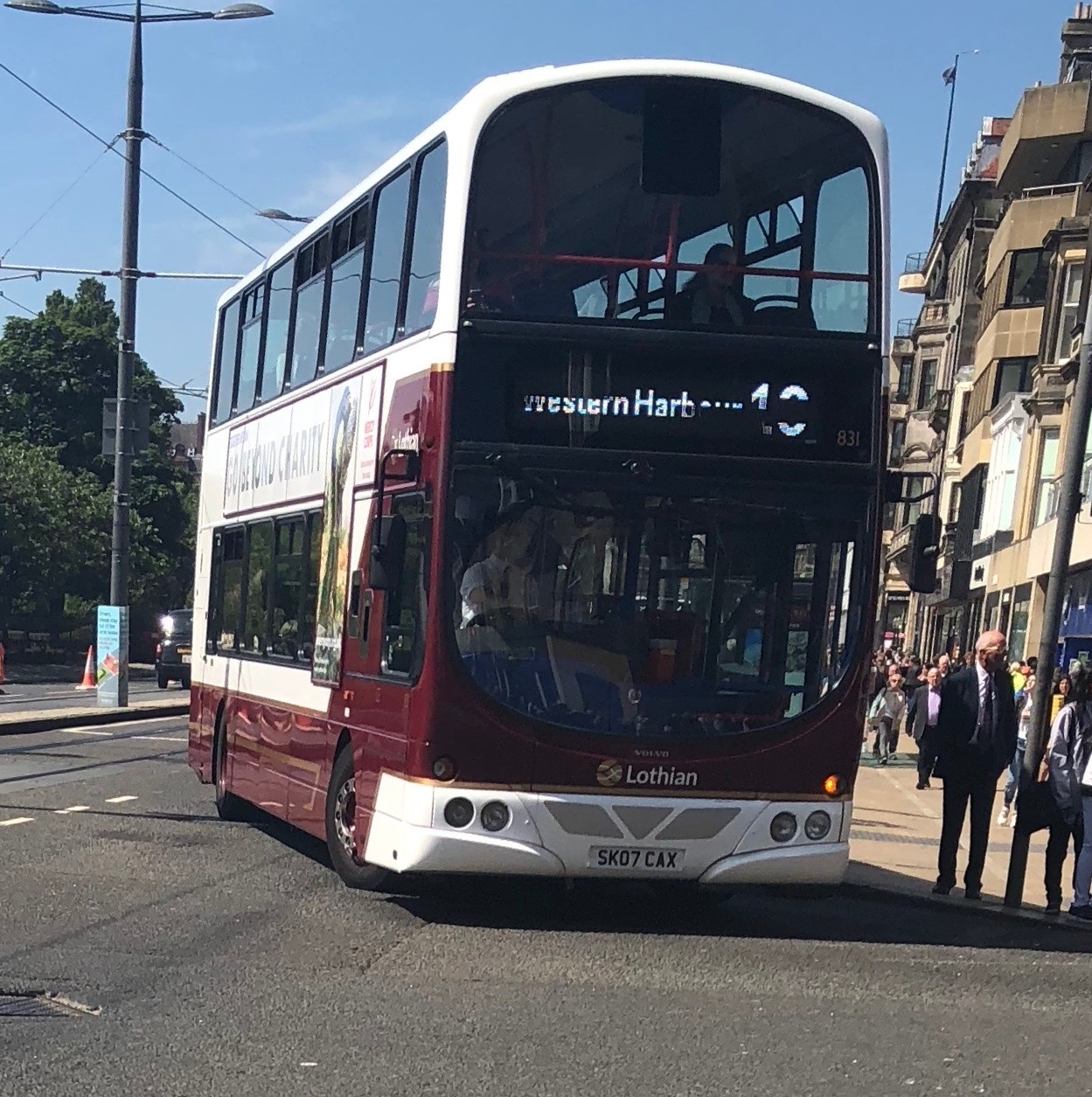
[156,609,193,690]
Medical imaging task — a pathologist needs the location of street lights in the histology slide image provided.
[3,0,273,708]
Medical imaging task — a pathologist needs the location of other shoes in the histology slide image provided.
[1010,809,1017,827]
[966,892,982,901]
[916,781,924,790]
[997,805,1010,824]
[881,757,887,764]
[924,778,930,789]
[1068,901,1092,921]
[1045,902,1064,914]
[932,880,956,894]
[889,752,898,760]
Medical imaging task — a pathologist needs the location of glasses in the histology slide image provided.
[891,679,902,683]
[982,645,1007,654]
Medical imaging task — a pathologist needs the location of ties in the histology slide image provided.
[983,673,993,742]
[502,567,511,599]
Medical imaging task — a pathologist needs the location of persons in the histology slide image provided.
[1038,661,1092,921]
[863,645,974,790]
[932,631,1018,899]
[675,244,755,327]
[481,214,589,315]
[458,522,539,627]
[997,657,1038,828]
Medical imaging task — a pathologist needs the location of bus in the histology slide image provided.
[188,59,942,889]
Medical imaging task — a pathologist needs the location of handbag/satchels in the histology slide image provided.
[1030,779,1054,832]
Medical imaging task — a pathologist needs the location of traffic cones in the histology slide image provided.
[75,645,97,688]
